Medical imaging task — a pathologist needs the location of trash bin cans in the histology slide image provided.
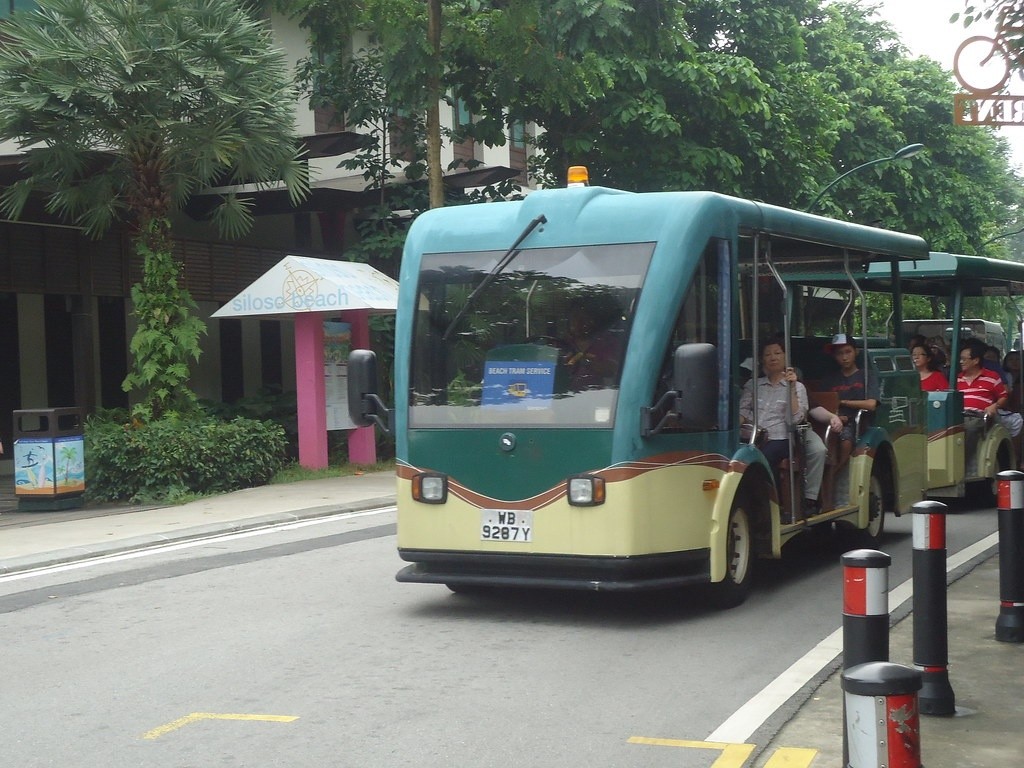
[12,406,86,513]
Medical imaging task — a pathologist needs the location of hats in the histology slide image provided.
[829,333,860,348]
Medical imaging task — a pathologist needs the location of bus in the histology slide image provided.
[396,164,1023,610]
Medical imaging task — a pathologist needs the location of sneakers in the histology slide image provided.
[780,512,800,524]
[801,497,824,517]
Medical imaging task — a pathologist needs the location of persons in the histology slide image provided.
[909,334,950,391]
[956,337,1023,470]
[819,333,881,469]
[557,294,624,392]
[738,340,842,519]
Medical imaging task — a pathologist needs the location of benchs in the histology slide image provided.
[781,390,847,517]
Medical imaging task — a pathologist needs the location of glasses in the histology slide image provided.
[912,353,928,357]
[960,356,975,362]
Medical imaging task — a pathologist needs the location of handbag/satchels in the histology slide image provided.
[738,420,769,448]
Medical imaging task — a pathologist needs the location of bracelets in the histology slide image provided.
[996,401,1000,409]
